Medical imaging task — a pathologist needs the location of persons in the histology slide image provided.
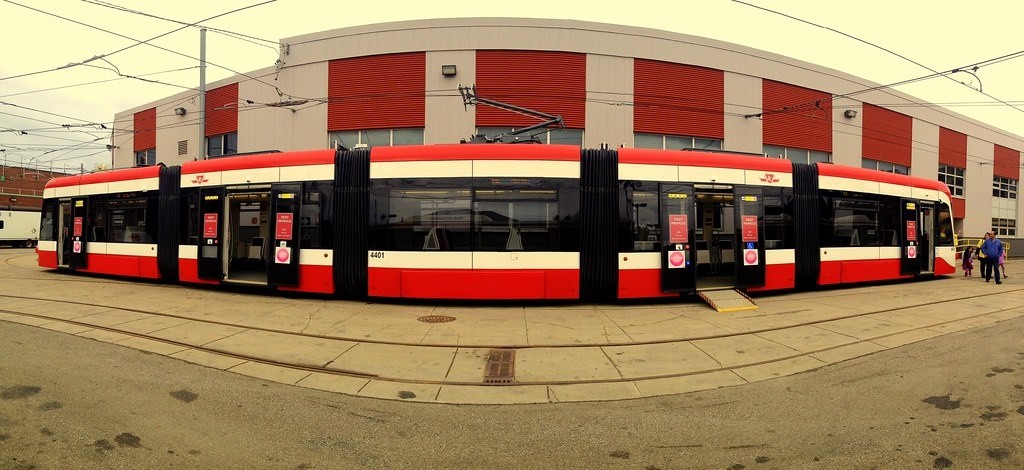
[963,246,974,276]
[976,233,993,279]
[999,247,1008,279]
[981,231,1001,284]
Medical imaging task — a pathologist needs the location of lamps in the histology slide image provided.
[845,110,858,118]
[442,64,456,76]
[174,107,187,115]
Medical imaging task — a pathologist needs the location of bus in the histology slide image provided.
[0,205,42,248]
[36,85,958,313]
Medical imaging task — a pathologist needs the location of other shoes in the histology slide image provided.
[969,273,972,275]
[965,272,968,276]
[1004,275,1008,279]
[982,276,986,278]
[996,281,1002,284]
[985,278,990,282]
[989,276,994,279]
